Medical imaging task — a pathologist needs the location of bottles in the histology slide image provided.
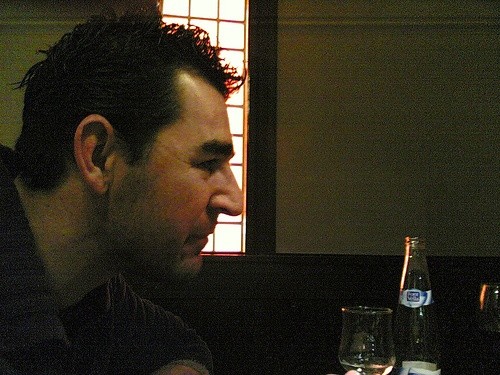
[395,236,438,370]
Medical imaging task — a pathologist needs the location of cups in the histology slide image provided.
[477,282,500,337]
[338,306,396,375]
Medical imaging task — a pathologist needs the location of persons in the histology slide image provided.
[0,5,244,375]
